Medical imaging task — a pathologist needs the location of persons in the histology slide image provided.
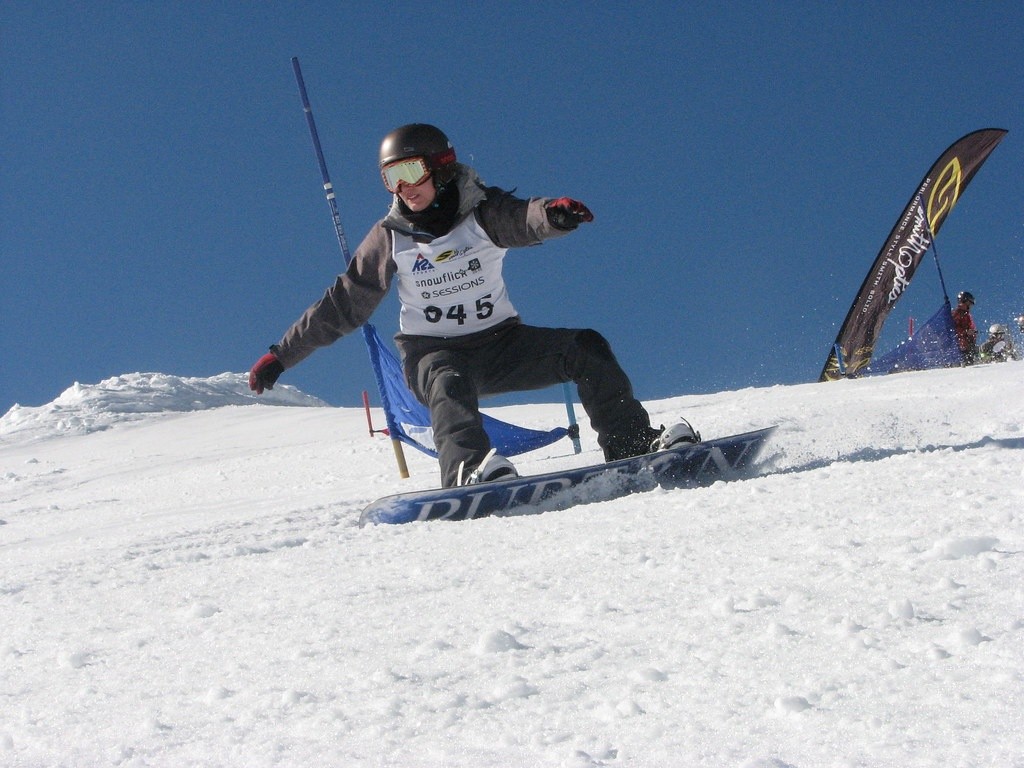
[246,124,697,488]
[951,289,978,365]
[1014,314,1024,336]
[979,322,1018,361]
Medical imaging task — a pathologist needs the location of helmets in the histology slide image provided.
[958,292,975,306]
[989,324,1005,334]
[378,123,456,171]
[1019,316,1024,332]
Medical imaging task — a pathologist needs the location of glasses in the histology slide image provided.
[380,155,433,193]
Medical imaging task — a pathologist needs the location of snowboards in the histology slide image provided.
[359,425,781,531]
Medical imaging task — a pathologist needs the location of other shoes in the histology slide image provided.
[621,426,698,458]
[470,454,519,485]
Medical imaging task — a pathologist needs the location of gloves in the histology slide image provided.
[249,351,284,394]
[547,197,593,230]
